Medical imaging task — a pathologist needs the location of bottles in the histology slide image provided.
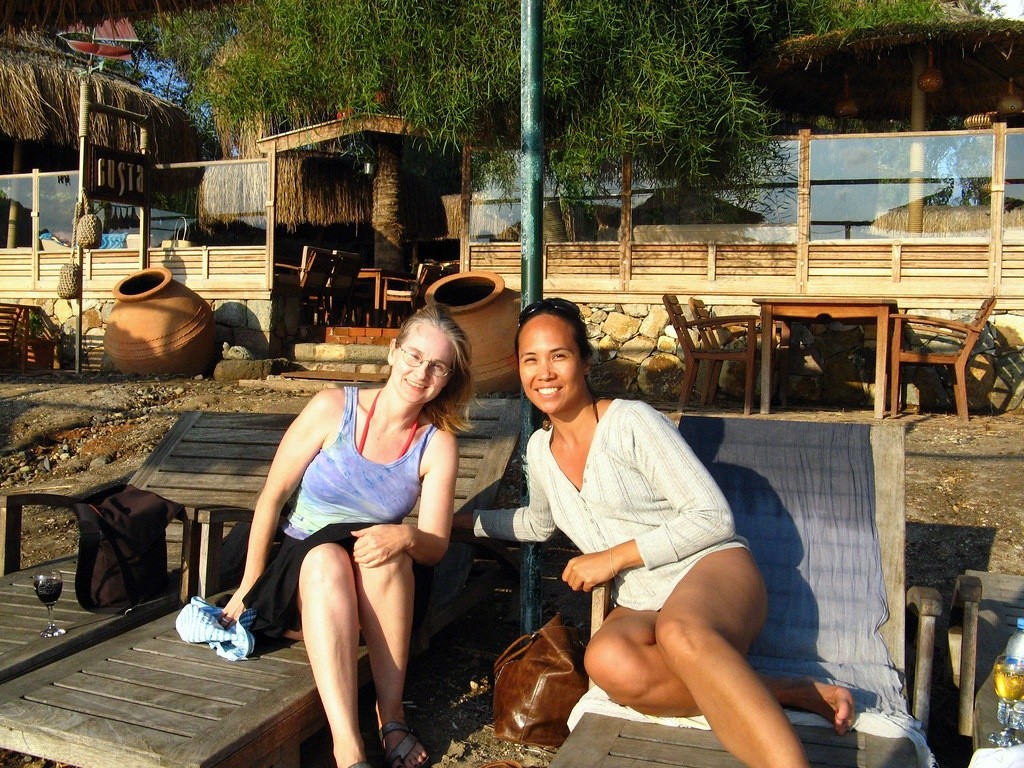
[996,617,1024,731]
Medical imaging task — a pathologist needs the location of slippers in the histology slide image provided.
[378,721,431,768]
[348,761,374,768]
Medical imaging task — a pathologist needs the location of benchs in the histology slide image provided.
[1,396,529,768]
[952,568,1024,754]
[2,409,312,683]
[548,418,945,766]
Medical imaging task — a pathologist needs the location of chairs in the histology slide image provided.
[273,248,459,324]
[689,297,758,406]
[889,296,998,422]
[663,294,760,415]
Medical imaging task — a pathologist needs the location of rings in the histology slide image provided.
[222,617,229,623]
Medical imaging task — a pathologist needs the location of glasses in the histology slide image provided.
[517,298,581,327]
[397,342,453,378]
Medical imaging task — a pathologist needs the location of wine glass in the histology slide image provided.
[32,570,66,638]
[988,654,1024,748]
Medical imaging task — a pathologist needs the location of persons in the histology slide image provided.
[219,301,489,768]
[453,296,854,768]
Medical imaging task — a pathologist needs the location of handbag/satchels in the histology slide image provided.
[487,613,589,748]
[71,484,185,614]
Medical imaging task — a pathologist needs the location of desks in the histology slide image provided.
[750,298,897,418]
[354,268,407,324]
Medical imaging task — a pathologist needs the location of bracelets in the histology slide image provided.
[610,547,616,579]
